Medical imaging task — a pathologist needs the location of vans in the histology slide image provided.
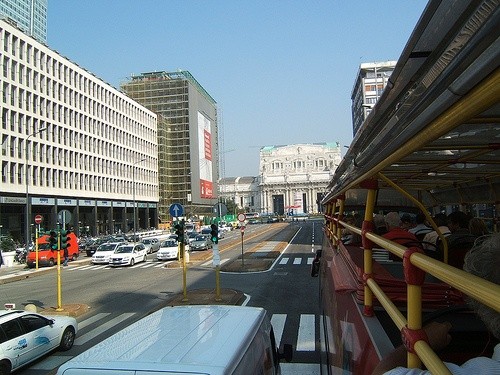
[28,232,79,267]
[57,305,293,375]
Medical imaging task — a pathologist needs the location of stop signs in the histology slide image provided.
[35,215,42,223]
[241,227,244,236]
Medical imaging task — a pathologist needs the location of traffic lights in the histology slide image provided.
[211,223,218,243]
[60,229,71,249]
[46,230,58,251]
[175,224,183,242]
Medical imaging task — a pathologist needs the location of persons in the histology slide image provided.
[370,231,500,375]
[63,248,68,266]
[335,209,500,375]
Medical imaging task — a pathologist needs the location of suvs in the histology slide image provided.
[0,309,78,375]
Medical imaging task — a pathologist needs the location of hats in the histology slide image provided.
[374,215,386,228]
[384,212,402,227]
[434,213,449,221]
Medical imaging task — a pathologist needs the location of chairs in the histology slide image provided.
[378,226,495,269]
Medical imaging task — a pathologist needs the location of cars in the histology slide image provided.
[266,214,308,224]
[248,219,258,224]
[80,222,242,267]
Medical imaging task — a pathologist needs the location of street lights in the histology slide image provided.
[26,127,47,240]
[132,159,147,243]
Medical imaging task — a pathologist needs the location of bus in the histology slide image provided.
[310,1,500,375]
